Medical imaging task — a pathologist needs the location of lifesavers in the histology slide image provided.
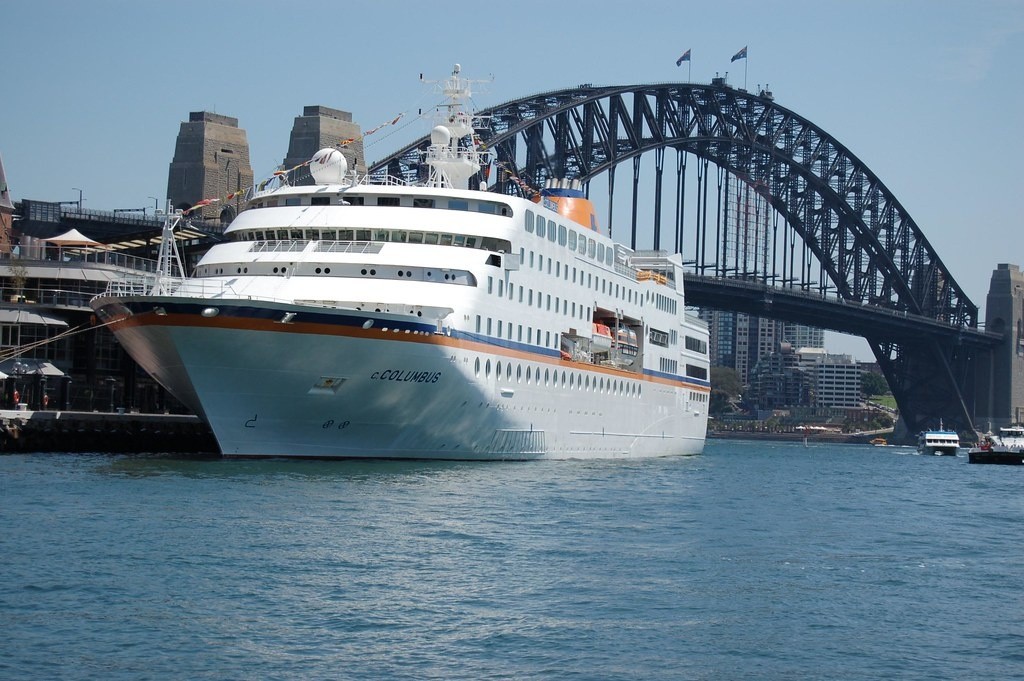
[44,395,48,404]
[14,390,20,403]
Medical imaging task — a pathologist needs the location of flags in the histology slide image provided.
[731,45,747,61]
[676,49,690,66]
[473,131,541,196]
[182,112,403,216]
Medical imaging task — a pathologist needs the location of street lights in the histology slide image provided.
[72,188,82,213]
[148,197,158,208]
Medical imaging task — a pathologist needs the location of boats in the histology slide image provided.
[968,422,1024,465]
[870,438,887,446]
[90,63,711,460]
[917,418,960,456]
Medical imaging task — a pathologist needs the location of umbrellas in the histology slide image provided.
[40,228,109,262]
